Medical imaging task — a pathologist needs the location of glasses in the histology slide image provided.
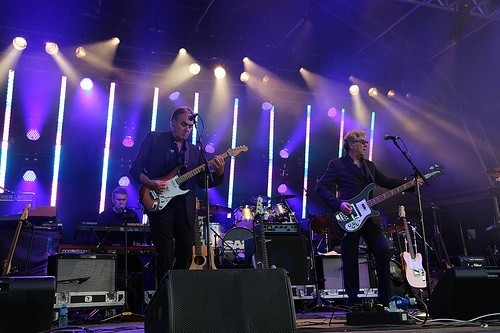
[352,139,368,144]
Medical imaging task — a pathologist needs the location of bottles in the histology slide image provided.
[58,304,68,327]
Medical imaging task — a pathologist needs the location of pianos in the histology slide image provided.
[56,219,159,325]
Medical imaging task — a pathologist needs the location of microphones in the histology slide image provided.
[188,113,198,121]
[384,134,401,140]
[238,256,244,262]
[120,205,126,212]
[78,276,91,284]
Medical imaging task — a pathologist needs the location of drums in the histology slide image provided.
[233,205,256,223]
[222,220,258,265]
[271,203,290,220]
[206,223,223,250]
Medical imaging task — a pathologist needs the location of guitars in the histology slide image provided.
[188,198,217,270]
[398,205,427,289]
[1,203,32,276]
[334,165,445,233]
[138,145,249,212]
[256,196,276,269]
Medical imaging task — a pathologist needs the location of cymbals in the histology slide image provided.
[264,194,297,200]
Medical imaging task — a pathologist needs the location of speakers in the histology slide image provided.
[314,256,377,290]
[0,232,309,333]
[426,256,500,323]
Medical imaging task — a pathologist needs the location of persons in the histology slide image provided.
[315,130,425,307]
[130,107,225,290]
[97,187,153,310]
